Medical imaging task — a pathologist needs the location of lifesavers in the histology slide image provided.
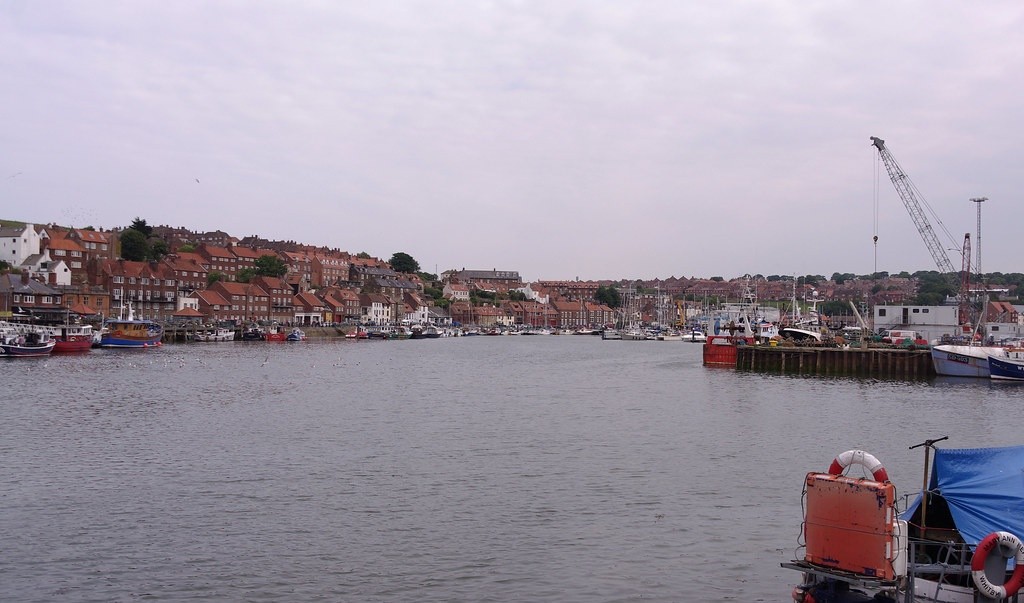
[829,450,889,483]
[972,532,1024,600]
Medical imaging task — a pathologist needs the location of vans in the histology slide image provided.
[879,330,916,348]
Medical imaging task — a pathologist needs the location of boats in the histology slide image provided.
[100,287,164,349]
[988,355,1024,383]
[192,270,791,360]
[780,291,834,345]
[780,434,1024,603]
[933,343,1024,377]
[0,309,56,358]
[44,301,95,353]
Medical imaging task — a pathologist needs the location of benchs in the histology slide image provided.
[905,536,973,599]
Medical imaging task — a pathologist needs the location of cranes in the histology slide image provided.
[868,137,1021,347]
